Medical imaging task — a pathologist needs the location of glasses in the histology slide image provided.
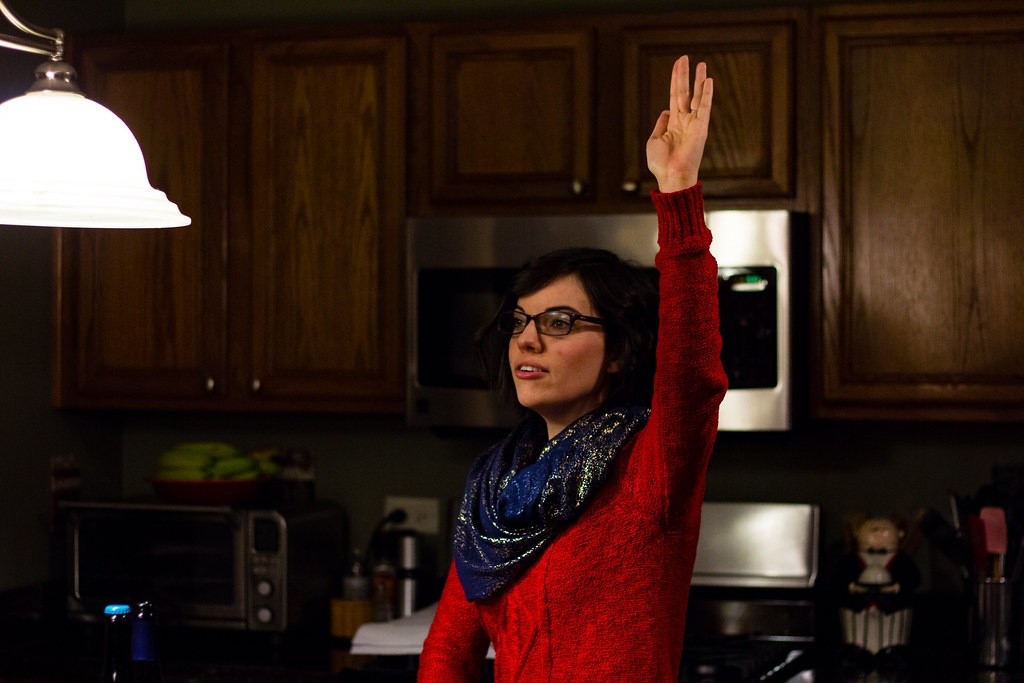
[498,310,605,336]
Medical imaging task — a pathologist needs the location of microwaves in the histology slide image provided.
[400,205,794,434]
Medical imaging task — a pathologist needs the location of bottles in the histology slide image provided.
[94,604,135,683]
[131,601,164,683]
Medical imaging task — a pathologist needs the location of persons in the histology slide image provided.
[420,54,726,683]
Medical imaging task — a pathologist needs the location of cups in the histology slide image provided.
[391,535,422,619]
[965,573,1017,668]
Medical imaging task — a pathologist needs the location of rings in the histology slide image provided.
[689,110,697,113]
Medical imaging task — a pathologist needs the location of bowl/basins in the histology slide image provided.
[142,476,275,501]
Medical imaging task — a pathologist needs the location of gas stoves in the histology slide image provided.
[677,501,823,683]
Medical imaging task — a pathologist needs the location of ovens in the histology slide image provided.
[56,492,349,636]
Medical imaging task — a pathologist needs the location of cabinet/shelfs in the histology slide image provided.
[806,0,1024,425]
[47,21,410,415]
[407,7,810,220]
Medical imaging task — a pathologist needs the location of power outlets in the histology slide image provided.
[383,495,440,535]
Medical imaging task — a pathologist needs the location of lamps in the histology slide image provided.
[0,0,192,229]
